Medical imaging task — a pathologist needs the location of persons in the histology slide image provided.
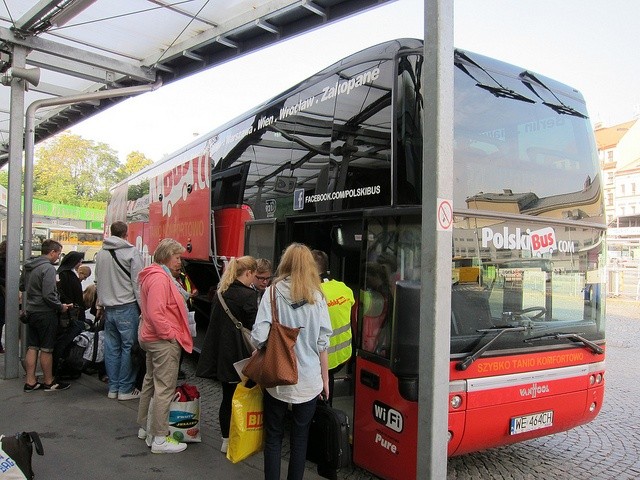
[250,257,273,308]
[0,240,22,354]
[94,221,143,401]
[135,238,193,454]
[311,250,356,480]
[56,251,86,379]
[249,243,333,480]
[18,241,68,393]
[169,263,199,378]
[196,256,258,453]
[75,285,110,382]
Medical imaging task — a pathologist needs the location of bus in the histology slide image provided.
[101,36,609,476]
[18,222,105,263]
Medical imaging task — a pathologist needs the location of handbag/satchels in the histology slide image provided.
[52,337,87,379]
[145,392,202,447]
[226,377,265,464]
[240,325,258,357]
[73,317,104,363]
[0,432,45,480]
[53,308,85,354]
[0,436,28,480]
[290,390,352,472]
[241,321,305,391]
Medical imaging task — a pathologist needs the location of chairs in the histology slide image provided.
[453,281,495,335]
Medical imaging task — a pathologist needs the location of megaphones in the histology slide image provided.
[11,67,40,87]
[2,76,29,92]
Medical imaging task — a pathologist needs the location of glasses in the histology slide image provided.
[255,275,273,283]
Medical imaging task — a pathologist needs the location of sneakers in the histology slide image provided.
[137,425,147,440]
[151,436,188,454]
[107,383,117,399]
[221,437,228,454]
[43,378,71,391]
[97,372,109,382]
[24,382,43,392]
[117,387,141,400]
